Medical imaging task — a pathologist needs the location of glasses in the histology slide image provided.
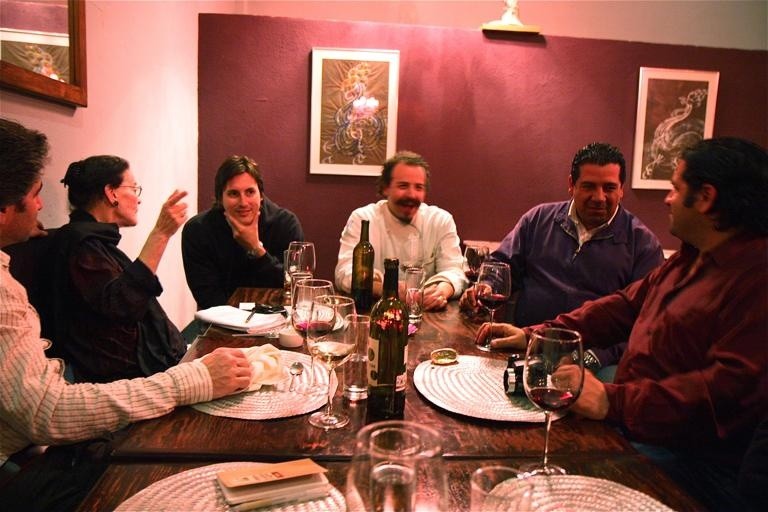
[120,184,142,196]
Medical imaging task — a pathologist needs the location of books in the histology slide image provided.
[214,455,333,511]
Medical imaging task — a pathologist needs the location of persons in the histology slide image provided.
[179,151,306,310]
[28,152,189,386]
[332,149,471,312]
[0,116,254,512]
[473,133,767,511]
[455,141,665,330]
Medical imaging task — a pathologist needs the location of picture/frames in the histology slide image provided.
[628,65,721,192]
[308,46,401,177]
[0,0,88,108]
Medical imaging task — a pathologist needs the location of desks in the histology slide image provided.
[112,281,639,461]
[75,460,704,512]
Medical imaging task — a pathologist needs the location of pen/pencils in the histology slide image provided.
[230,487,322,512]
[246,310,256,323]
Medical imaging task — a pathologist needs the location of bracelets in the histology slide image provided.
[246,240,264,260]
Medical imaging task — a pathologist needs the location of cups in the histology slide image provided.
[347,420,451,512]
[405,266,427,321]
[469,465,536,512]
[343,313,368,401]
[368,428,418,512]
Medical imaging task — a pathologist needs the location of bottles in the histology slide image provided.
[353,219,374,312]
[365,255,409,425]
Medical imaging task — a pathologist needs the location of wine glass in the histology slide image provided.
[476,261,511,352]
[286,240,357,429]
[520,328,585,477]
[463,245,492,318]
